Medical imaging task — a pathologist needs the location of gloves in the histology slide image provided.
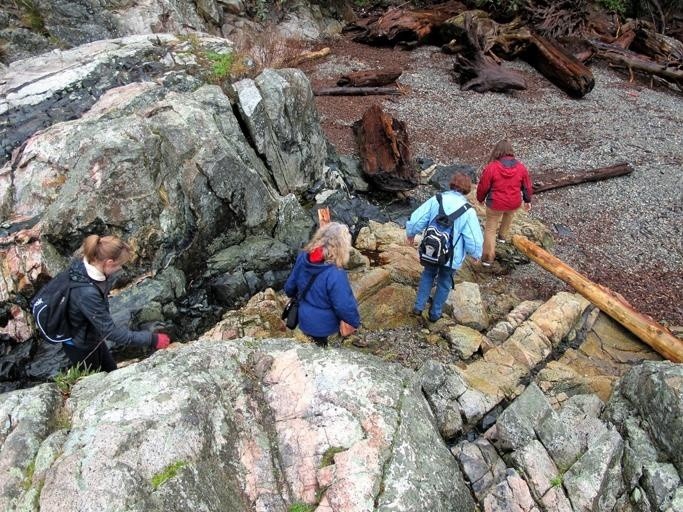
[156,333,170,349]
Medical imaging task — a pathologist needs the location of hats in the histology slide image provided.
[448,173,471,194]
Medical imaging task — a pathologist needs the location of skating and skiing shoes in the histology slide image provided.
[481,237,506,267]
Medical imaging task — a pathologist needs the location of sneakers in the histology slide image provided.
[411,307,442,322]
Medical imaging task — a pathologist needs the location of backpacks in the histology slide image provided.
[418,193,472,268]
[30,267,106,346]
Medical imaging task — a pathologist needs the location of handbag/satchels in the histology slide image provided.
[280,296,299,330]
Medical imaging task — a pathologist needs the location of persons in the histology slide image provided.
[475,139,534,267]
[406,171,482,321]
[281,220,360,350]
[61,235,170,372]
